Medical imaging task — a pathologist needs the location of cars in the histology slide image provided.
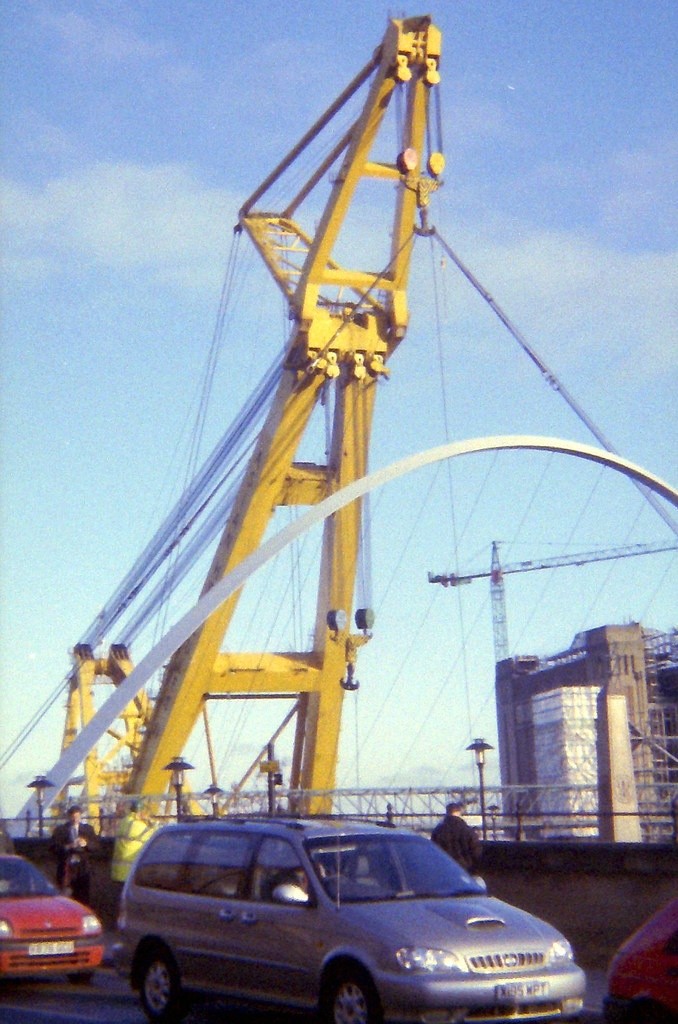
[602,896,678,1024]
[0,855,103,984]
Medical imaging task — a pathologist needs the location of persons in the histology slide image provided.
[108,799,158,906]
[50,806,99,907]
[286,849,326,890]
[431,803,482,871]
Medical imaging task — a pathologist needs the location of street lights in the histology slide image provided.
[465,738,492,841]
[27,775,57,840]
[486,804,502,842]
[203,784,224,819]
[164,756,196,823]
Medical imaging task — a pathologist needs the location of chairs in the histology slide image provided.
[251,866,276,900]
[350,855,379,888]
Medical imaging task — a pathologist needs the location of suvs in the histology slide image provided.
[114,818,587,1023]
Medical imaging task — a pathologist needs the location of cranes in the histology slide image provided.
[428,532,678,669]
